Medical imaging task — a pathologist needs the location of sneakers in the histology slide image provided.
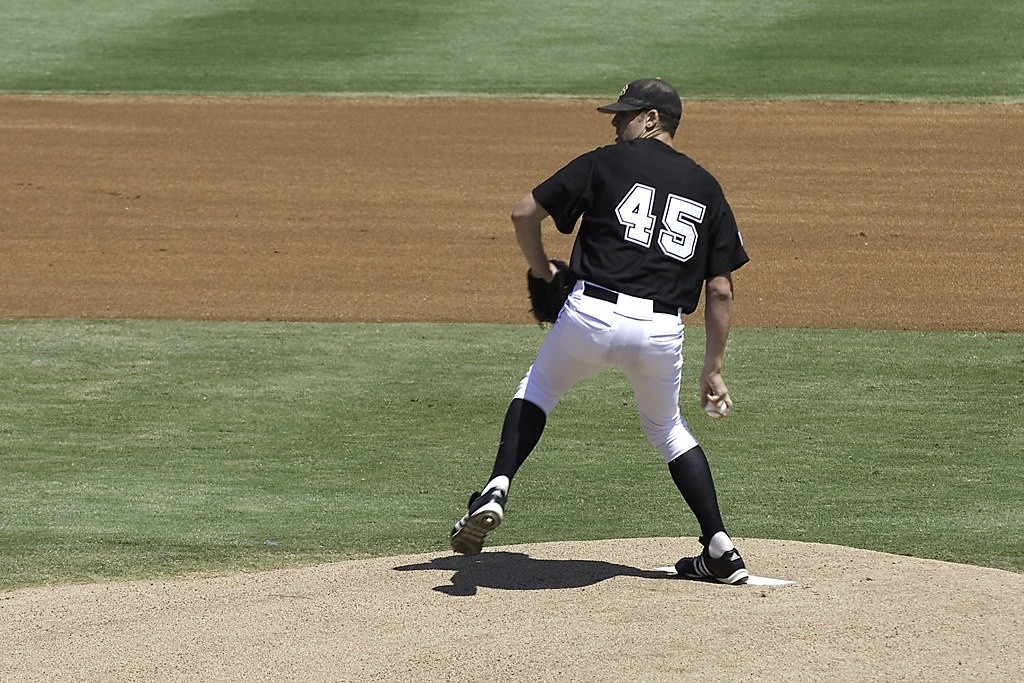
[450,487,506,556]
[674,548,749,584]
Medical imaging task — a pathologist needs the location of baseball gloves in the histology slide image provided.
[525,259,573,324]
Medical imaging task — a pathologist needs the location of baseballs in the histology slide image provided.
[705,396,728,419]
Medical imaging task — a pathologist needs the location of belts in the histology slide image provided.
[583,283,678,316]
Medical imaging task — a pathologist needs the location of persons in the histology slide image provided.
[450,79,752,584]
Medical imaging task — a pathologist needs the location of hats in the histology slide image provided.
[597,79,682,114]
